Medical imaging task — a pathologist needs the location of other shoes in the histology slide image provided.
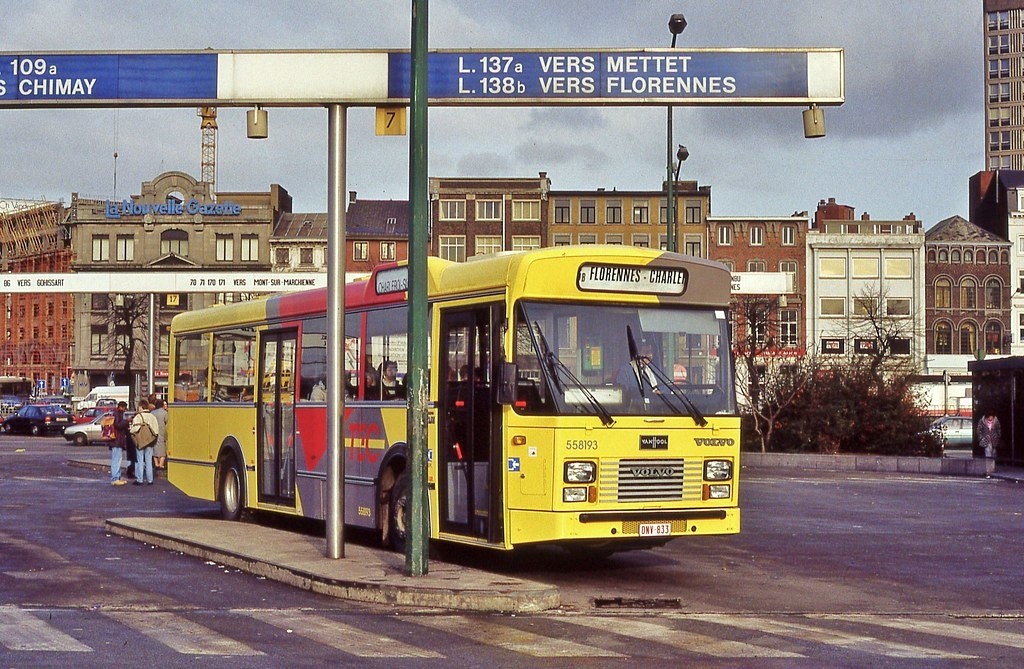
[120,480,128,484]
[155,466,159,469]
[111,480,124,486]
[133,480,143,485]
[159,466,167,470]
[148,482,153,485]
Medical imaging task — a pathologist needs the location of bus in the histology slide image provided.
[166,243,742,563]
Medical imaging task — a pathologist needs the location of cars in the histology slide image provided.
[1,405,76,437]
[77,407,133,424]
[64,412,135,446]
[929,417,973,448]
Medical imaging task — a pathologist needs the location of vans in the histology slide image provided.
[77,386,129,411]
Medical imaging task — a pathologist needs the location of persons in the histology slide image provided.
[977,410,1000,459]
[447,365,490,413]
[109,393,167,486]
[616,342,671,395]
[310,361,408,402]
[176,365,254,403]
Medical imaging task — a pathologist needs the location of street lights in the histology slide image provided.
[674,148,689,252]
[667,14,688,252]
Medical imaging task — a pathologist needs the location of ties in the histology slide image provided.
[641,363,653,400]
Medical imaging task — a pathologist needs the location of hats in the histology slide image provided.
[118,401,129,411]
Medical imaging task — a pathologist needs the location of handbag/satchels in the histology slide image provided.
[100,414,117,441]
[130,412,159,451]
